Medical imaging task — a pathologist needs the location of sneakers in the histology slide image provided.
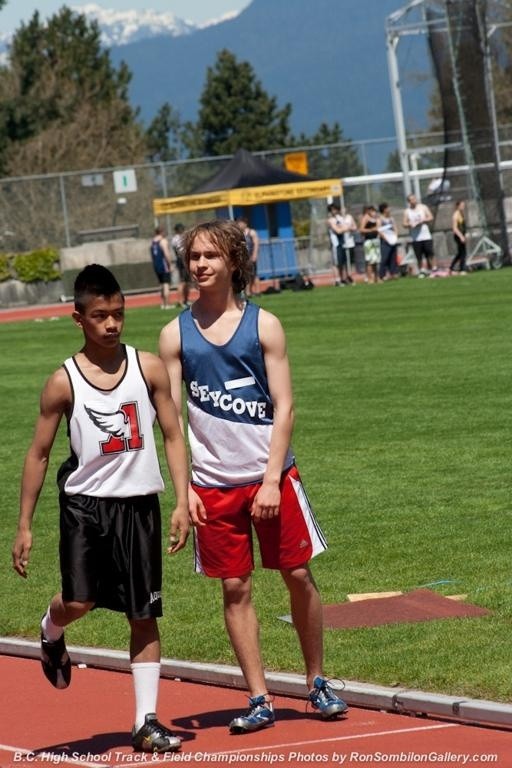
[305,675,348,717]
[41,613,71,689]
[229,692,276,731]
[335,268,427,288]
[160,301,192,310]
[131,713,181,753]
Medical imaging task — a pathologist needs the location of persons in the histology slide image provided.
[236,216,259,298]
[377,201,400,281]
[12,264,192,753]
[339,205,357,288]
[149,224,177,310]
[326,202,348,288]
[171,221,191,309]
[448,200,469,276]
[359,205,384,285]
[403,194,438,280]
[156,218,349,734]
